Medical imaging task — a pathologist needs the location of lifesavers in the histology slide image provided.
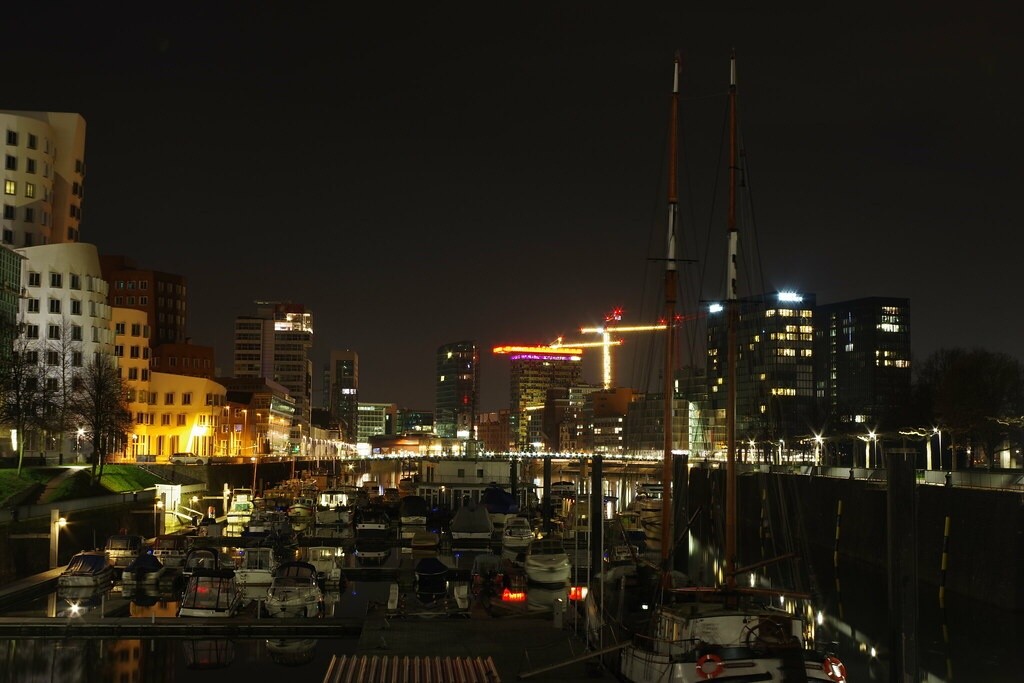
[825,657,846,680]
[694,653,722,678]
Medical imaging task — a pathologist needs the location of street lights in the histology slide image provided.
[224,405,304,458]
[930,426,943,470]
[814,436,826,466]
[866,432,879,468]
[778,439,786,465]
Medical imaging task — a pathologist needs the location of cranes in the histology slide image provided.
[578,324,668,390]
[657,310,712,371]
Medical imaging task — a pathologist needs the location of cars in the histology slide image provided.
[168,451,208,464]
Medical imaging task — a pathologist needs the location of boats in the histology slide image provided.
[264,637,320,666]
[51,467,674,620]
[181,640,236,669]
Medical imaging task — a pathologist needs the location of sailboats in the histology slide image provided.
[579,58,846,683]
